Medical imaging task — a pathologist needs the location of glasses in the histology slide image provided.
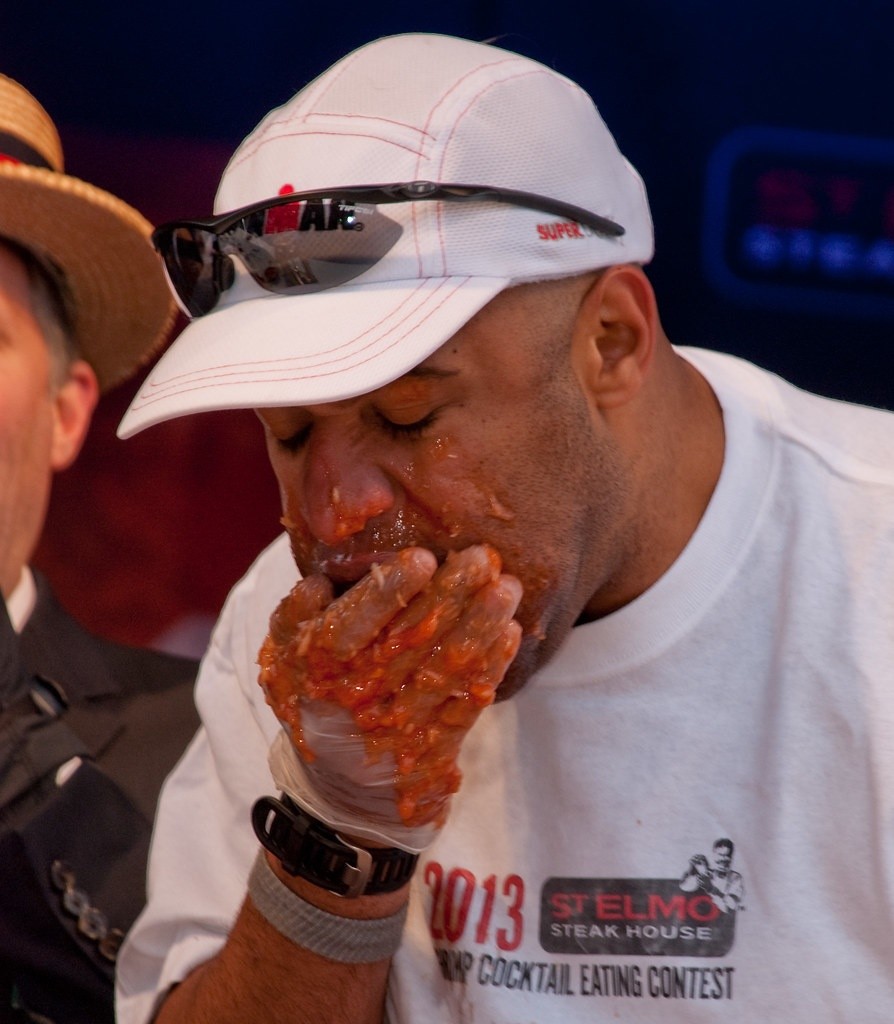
[150,179,625,317]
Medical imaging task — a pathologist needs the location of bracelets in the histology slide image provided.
[250,794,419,900]
[245,848,408,963]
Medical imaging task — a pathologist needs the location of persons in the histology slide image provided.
[0,70,210,1024]
[109,28,894,1024]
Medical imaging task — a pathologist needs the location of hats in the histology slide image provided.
[115,33,654,440]
[0,67,179,395]
